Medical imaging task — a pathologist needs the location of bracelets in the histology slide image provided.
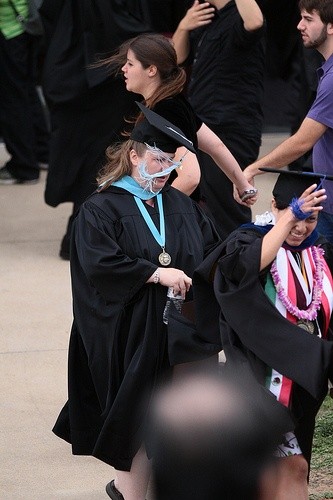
[289,199,313,220]
[153,266,159,284]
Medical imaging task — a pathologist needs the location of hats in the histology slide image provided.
[258,168,333,220]
[130,101,196,194]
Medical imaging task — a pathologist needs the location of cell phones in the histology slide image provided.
[241,189,257,202]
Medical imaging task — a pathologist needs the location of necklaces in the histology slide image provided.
[270,244,325,321]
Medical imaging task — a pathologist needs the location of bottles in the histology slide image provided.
[162,286,185,325]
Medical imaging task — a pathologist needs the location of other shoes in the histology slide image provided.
[105,478,147,500]
[0,167,39,185]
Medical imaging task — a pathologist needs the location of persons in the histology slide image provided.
[0,0,333,500]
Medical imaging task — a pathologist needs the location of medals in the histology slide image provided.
[158,251,171,266]
